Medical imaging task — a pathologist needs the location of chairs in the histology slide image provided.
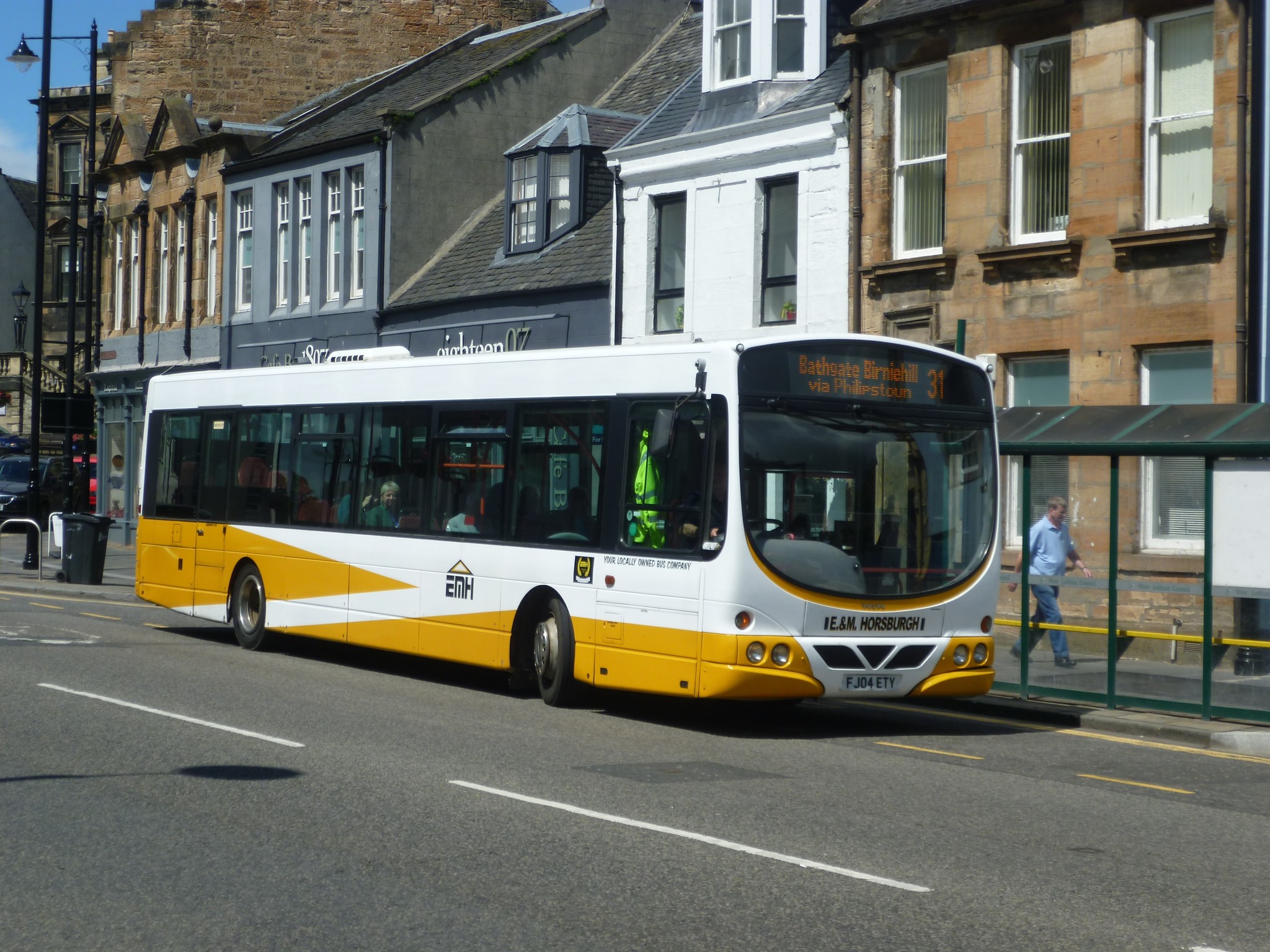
[238,455,452,531]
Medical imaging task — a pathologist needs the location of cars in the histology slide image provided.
[72,457,99,508]
[0,453,66,530]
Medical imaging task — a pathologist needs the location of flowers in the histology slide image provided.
[72,428,97,442]
[0,388,12,408]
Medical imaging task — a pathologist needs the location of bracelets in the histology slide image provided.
[1082,567,1088,571]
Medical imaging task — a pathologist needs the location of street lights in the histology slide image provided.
[13,280,32,352]
[4,23,101,512]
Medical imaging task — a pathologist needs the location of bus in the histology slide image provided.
[133,337,1006,710]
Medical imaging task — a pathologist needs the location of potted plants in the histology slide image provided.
[781,299,797,321]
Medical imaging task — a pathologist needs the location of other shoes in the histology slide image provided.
[1054,657,1077,668]
[1009,646,1034,663]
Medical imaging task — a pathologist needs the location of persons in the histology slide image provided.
[1007,497,1092,668]
[338,471,597,543]
[666,460,752,553]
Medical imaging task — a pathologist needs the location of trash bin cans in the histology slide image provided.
[55,513,117,585]
